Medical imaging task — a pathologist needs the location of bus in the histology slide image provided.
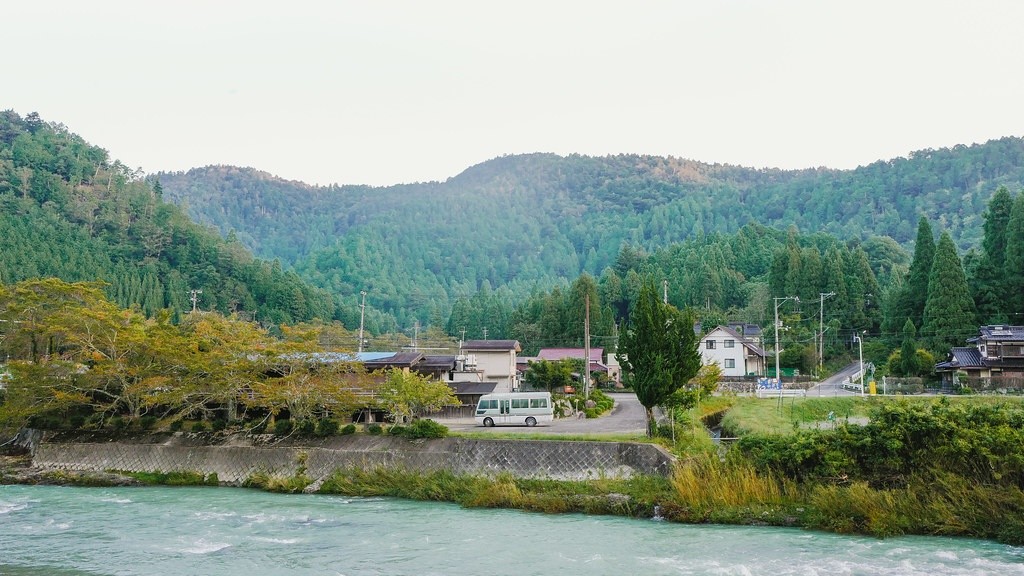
[474,392,552,427]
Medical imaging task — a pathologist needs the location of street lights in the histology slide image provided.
[855,337,864,395]
